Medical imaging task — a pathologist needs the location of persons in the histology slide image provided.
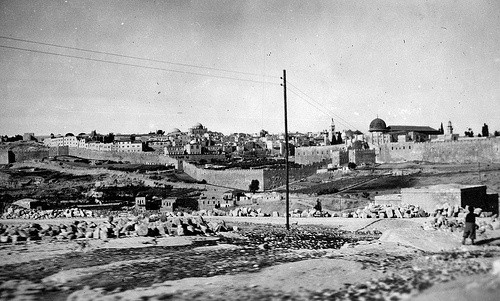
[462,206,483,245]
[312,198,323,217]
